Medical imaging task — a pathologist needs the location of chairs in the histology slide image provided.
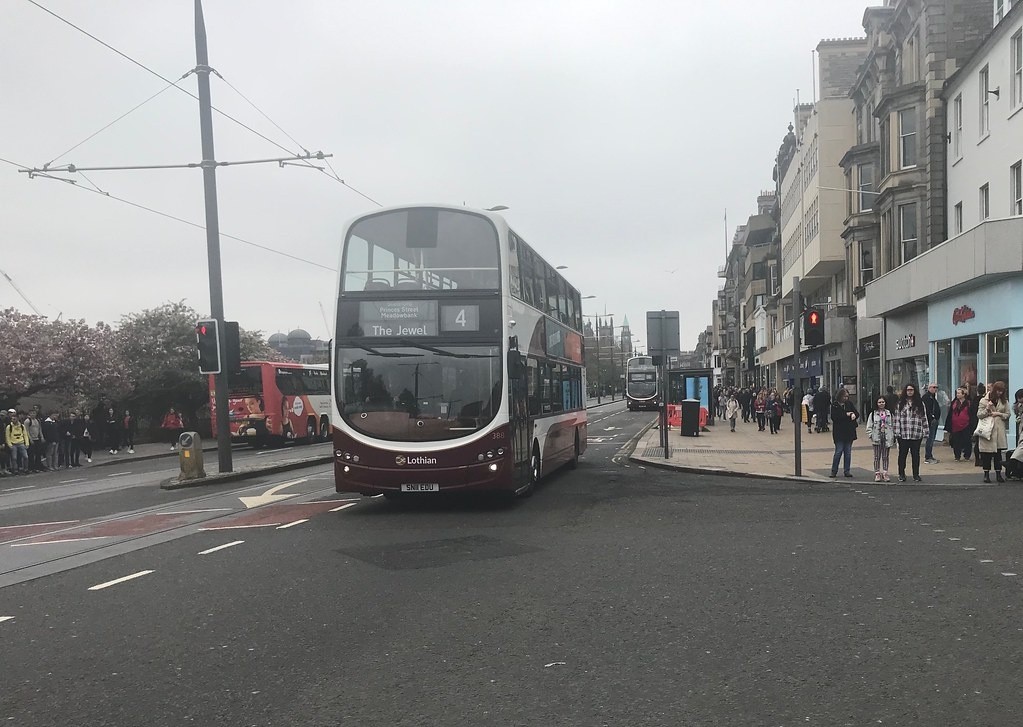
[363,278,422,290]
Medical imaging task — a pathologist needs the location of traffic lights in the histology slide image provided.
[804,309,826,346]
[194,319,222,372]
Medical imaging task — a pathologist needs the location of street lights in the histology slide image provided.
[581,311,646,403]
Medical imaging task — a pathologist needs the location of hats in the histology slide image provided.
[0,408,16,416]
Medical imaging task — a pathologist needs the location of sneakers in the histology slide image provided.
[875,471,881,482]
[882,471,890,481]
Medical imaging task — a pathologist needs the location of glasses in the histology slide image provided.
[933,386,939,389]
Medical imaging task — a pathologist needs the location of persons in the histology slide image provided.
[160,408,185,451]
[279,396,297,439]
[884,386,899,445]
[237,394,272,436]
[922,383,941,464]
[892,384,930,482]
[829,388,859,478]
[0,403,97,477]
[865,396,894,482]
[943,380,1023,483]
[122,409,135,454]
[105,407,122,455]
[713,383,844,435]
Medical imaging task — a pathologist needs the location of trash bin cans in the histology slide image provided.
[680,399,700,437]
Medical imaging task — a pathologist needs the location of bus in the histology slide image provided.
[208,359,334,447]
[626,356,664,412]
[329,204,590,505]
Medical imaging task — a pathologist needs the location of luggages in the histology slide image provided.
[1006,420,1019,476]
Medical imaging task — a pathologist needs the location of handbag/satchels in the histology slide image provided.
[973,399,995,440]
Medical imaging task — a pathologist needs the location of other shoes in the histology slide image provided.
[924,458,940,464]
[807,426,821,434]
[109,449,119,455]
[898,475,906,482]
[829,471,836,478]
[844,472,853,477]
[913,475,922,482]
[170,446,178,451]
[127,448,134,454]
[996,471,1004,482]
[84,455,93,462]
[984,471,990,482]
